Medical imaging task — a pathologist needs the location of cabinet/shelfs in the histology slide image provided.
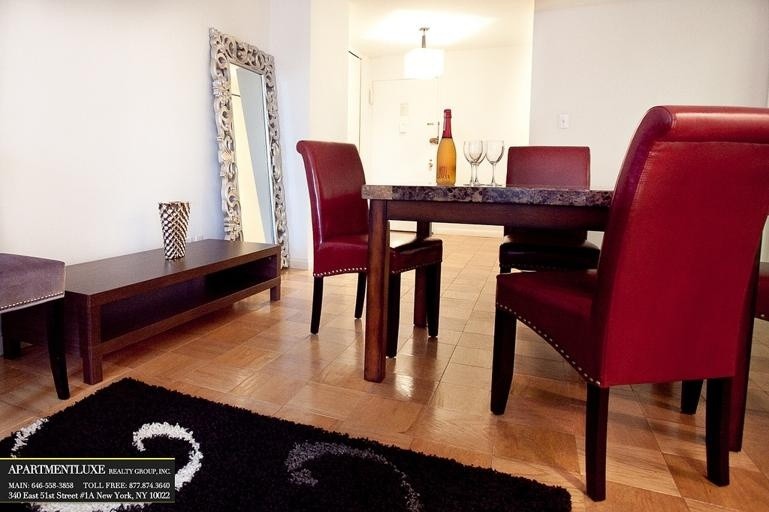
[64,237,283,386]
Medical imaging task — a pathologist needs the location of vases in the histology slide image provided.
[157,199,192,262]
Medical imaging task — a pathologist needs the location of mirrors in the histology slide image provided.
[209,25,291,271]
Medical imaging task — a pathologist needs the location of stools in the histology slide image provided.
[0,252,71,401]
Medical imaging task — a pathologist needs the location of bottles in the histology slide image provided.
[436,108,456,186]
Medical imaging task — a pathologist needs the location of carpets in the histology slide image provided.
[0,372,574,512]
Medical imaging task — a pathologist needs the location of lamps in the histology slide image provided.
[403,23,447,83]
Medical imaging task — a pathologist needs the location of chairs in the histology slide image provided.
[295,138,446,358]
[499,145,601,274]
[487,102,768,503]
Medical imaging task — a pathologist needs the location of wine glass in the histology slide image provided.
[463,138,505,187]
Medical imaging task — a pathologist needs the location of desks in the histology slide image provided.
[357,179,763,452]
